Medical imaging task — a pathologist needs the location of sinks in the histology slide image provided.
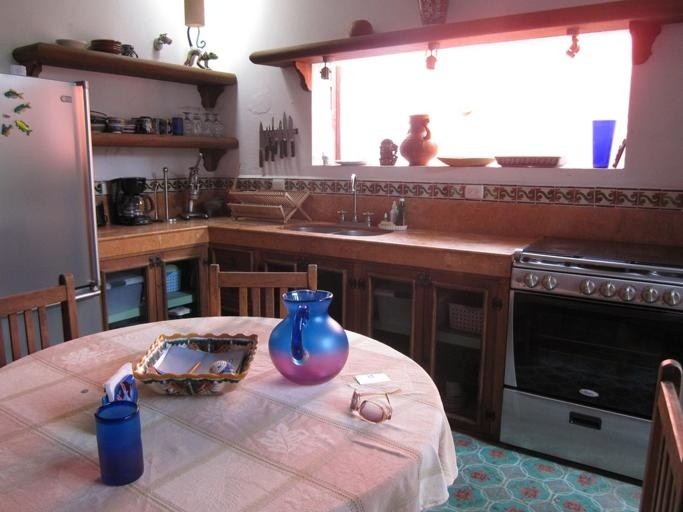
[277,223,341,232]
[327,228,393,236]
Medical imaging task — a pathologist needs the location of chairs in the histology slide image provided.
[639,359,683,512]
[0,272,80,369]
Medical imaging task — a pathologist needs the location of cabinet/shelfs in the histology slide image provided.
[208,224,508,441]
[11,42,239,172]
[100,228,210,330]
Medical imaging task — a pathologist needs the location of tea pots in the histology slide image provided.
[268,289,349,385]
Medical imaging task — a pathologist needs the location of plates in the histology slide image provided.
[438,156,560,167]
[55,40,123,54]
[336,161,367,166]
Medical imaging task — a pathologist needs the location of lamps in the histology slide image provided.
[184,0,206,48]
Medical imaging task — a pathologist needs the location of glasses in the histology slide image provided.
[349,390,398,424]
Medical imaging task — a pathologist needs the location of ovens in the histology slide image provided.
[499,291,683,485]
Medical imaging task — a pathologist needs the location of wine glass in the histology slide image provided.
[183,112,224,135]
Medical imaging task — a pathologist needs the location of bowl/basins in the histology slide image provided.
[90,111,134,135]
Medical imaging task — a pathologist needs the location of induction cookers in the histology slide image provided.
[508,235,682,312]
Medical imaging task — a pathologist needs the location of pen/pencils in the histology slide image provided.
[189,361,201,373]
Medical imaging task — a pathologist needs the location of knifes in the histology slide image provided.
[259,112,296,167]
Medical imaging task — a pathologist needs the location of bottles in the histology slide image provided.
[390,198,407,226]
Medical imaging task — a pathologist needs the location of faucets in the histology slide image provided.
[348,172,359,224]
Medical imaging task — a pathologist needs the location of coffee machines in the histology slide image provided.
[106,176,154,225]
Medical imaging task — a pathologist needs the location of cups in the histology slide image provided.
[94,401,143,487]
[136,116,182,135]
[592,120,616,168]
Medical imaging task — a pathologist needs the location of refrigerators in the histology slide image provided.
[0,75,100,370]
